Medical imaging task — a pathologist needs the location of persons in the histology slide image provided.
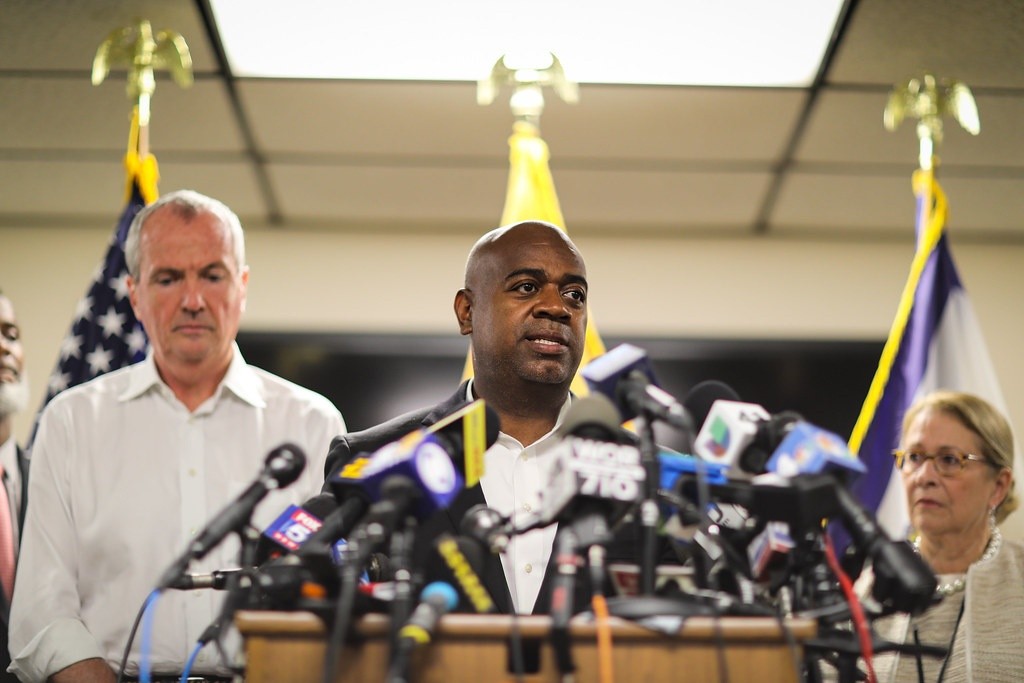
[819,392,1024,683]
[8,189,347,683]
[325,220,646,615]
[0,288,31,683]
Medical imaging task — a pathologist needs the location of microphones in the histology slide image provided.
[160,342,939,650]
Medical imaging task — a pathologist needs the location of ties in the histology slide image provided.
[0,464,16,602]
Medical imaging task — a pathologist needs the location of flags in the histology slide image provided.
[822,172,1024,562]
[459,129,632,430]
[24,150,161,446]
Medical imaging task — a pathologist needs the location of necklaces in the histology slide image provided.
[913,523,1001,595]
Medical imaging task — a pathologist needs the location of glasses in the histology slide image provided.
[891,447,986,477]
